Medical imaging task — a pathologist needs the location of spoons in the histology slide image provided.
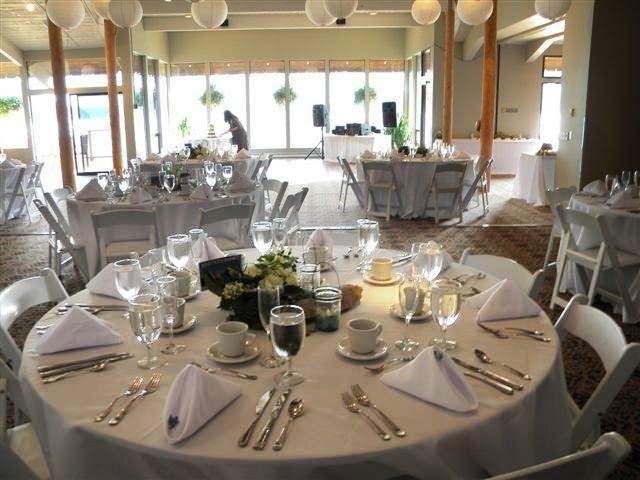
[273,398,306,450]
[474,349,533,379]
[42,358,110,384]
[344,247,353,256]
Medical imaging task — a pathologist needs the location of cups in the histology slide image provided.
[399,281,432,314]
[411,241,444,288]
[371,257,393,281]
[224,251,247,275]
[604,170,640,201]
[215,322,258,357]
[300,246,334,288]
[311,287,342,333]
[251,218,287,254]
[348,319,383,354]
[409,141,455,159]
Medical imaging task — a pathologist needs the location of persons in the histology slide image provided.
[219,110,249,152]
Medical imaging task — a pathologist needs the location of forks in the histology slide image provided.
[477,322,550,342]
[189,361,256,379]
[451,271,486,286]
[363,357,414,371]
[93,374,162,430]
[341,392,391,441]
[349,383,407,436]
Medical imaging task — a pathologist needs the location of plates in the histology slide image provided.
[389,303,433,322]
[336,336,389,362]
[208,342,260,365]
[363,274,402,286]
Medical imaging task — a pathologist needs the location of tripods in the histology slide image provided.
[304,127,325,161]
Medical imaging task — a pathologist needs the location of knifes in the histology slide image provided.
[450,355,523,390]
[254,388,292,451]
[40,351,134,378]
[238,387,275,446]
[56,303,132,312]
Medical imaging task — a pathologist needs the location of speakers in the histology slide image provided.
[382,101,396,128]
[312,104,328,126]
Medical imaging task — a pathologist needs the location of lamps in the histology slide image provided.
[188,1,230,30]
[106,0,145,29]
[324,0,359,21]
[456,0,495,26]
[532,0,573,20]
[304,1,335,29]
[411,0,442,25]
[46,0,87,30]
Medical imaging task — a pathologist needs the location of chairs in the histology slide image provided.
[337,144,493,224]
[0,354,51,479]
[2,156,46,224]
[540,176,640,323]
[37,135,308,284]
[0,267,73,424]
[554,292,639,451]
[475,430,632,480]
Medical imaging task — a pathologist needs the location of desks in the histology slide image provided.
[513,152,557,207]
[324,136,392,163]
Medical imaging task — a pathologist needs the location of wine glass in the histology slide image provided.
[257,285,288,367]
[96,141,238,206]
[269,305,308,390]
[428,279,461,349]
[356,219,379,273]
[113,229,208,369]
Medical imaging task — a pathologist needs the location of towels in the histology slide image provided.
[380,342,478,416]
[160,356,244,446]
[27,303,120,359]
